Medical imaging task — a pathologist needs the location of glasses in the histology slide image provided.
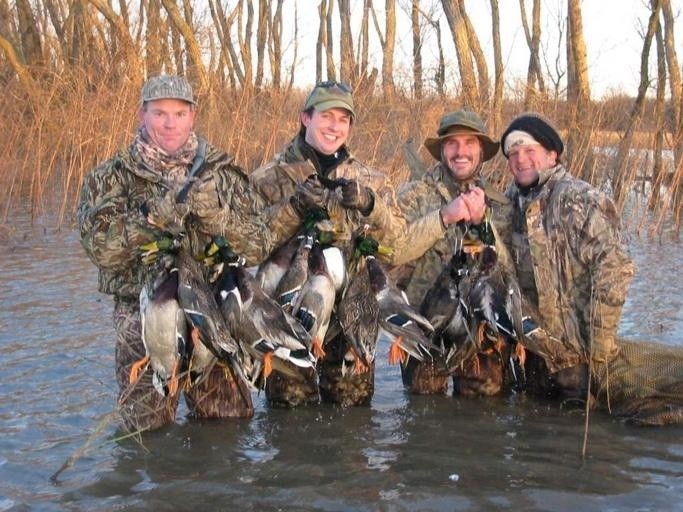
[316,80,352,92]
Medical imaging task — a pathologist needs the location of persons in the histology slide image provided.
[488,110,634,409]
[384,110,523,398]
[78,74,272,432]
[247,81,409,410]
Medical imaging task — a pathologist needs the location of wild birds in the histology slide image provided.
[130,207,527,397]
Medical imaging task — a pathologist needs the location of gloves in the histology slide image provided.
[157,188,192,220]
[188,170,221,221]
[289,175,328,219]
[340,179,371,216]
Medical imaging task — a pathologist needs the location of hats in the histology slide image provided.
[423,109,502,162]
[500,112,564,158]
[304,83,355,119]
[140,75,195,107]
